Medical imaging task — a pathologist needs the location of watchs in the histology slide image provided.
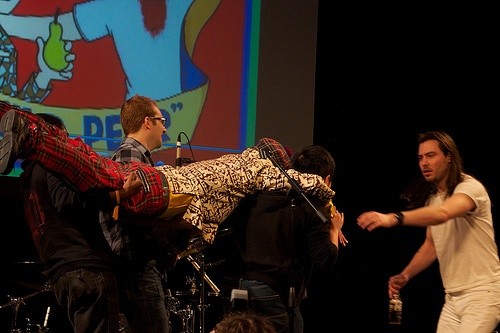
[394,211,404,225]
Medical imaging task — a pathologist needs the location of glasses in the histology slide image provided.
[151,117,168,126]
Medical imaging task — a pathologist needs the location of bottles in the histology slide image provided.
[389,295,403,324]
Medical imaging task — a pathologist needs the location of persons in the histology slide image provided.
[0,100,349,249]
[209,313,280,333]
[356,131,500,333]
[227,145,342,333]
[100,93,171,333]
[18,113,144,333]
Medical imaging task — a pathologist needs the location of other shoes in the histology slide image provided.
[1,108,28,175]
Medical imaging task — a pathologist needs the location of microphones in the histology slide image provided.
[176,133,181,170]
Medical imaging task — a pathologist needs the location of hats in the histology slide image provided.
[255,135,293,168]
